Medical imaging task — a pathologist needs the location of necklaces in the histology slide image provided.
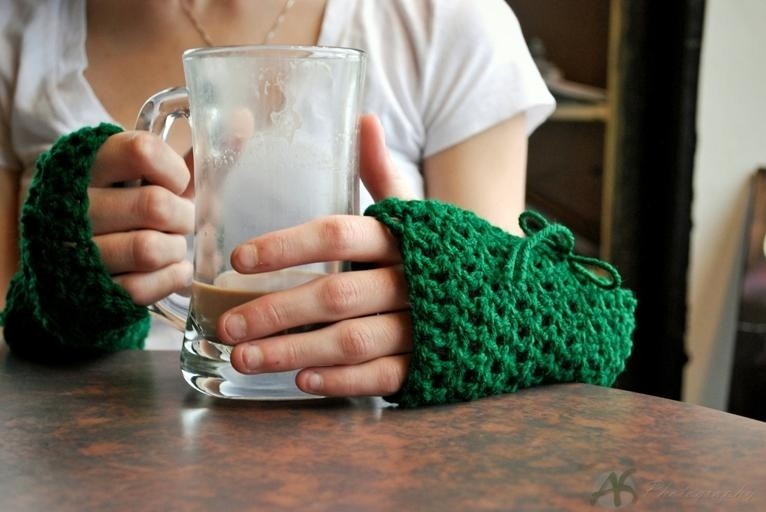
[176,0,293,46]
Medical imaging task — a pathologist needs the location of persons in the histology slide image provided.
[0,0,645,410]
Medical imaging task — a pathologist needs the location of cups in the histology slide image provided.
[127,42,369,407]
